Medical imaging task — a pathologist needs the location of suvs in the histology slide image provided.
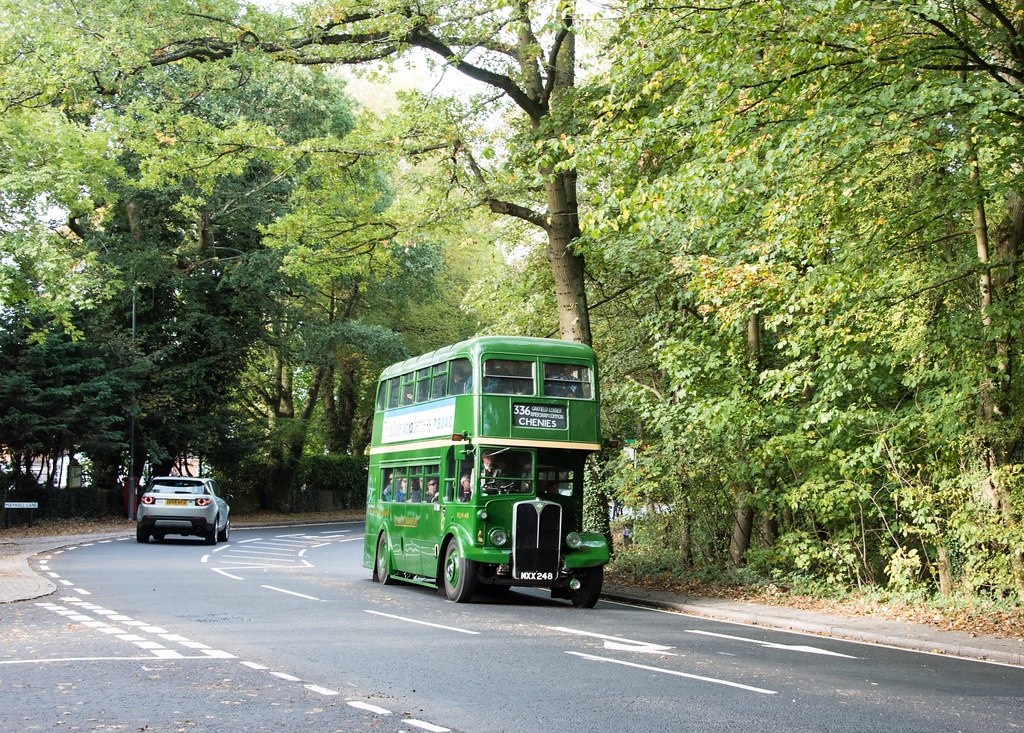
[135,475,235,546]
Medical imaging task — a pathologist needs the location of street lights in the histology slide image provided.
[127,282,146,522]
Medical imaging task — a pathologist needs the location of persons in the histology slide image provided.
[611,497,624,519]
[390,358,584,408]
[382,452,505,504]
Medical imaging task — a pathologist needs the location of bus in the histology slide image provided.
[360,335,620,609]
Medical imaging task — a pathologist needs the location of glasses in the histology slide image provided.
[429,482,438,486]
[390,478,392,481]
